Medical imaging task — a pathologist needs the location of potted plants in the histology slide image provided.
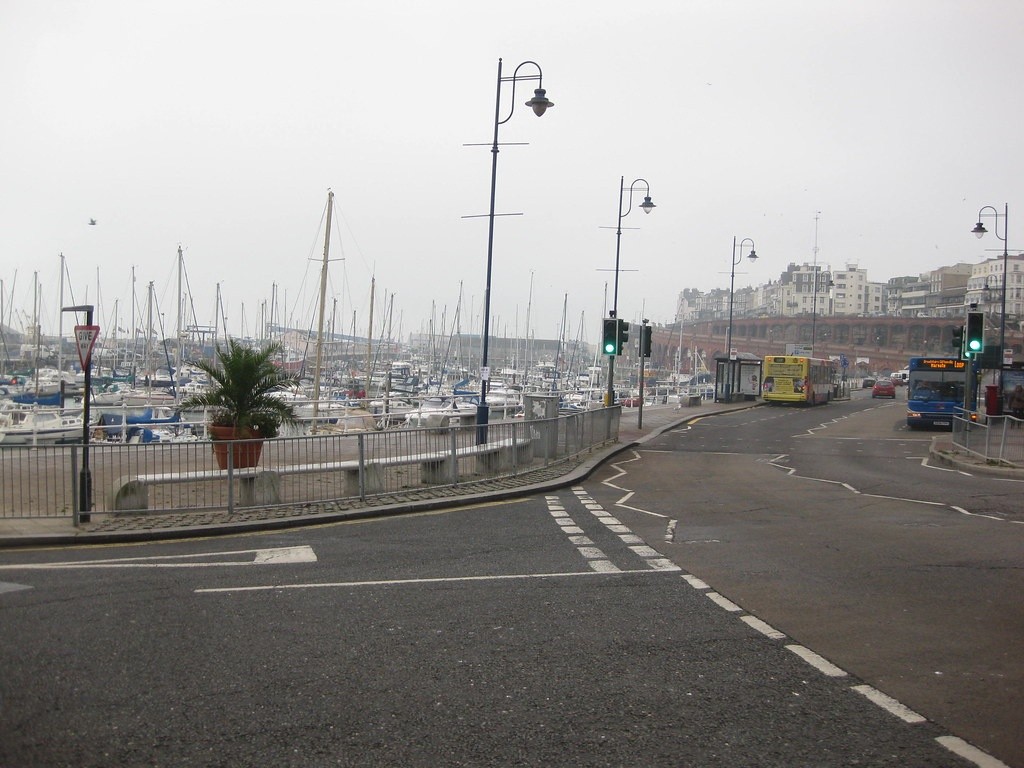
[173,335,304,470]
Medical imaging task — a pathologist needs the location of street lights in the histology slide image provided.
[811,267,836,357]
[725,236,759,403]
[983,274,1006,389]
[605,175,657,439]
[971,202,1008,416]
[475,57,555,446]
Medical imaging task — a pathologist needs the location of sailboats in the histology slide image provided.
[0,192,714,447]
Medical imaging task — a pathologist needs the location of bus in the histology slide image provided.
[761,356,836,405]
[906,356,977,431]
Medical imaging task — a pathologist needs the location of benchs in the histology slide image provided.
[589,401,621,418]
[436,444,505,485]
[681,394,701,407]
[266,460,360,504]
[364,453,447,495]
[105,468,255,518]
[425,413,477,433]
[488,436,533,469]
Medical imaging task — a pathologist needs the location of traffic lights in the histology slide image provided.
[964,311,985,354]
[952,326,964,349]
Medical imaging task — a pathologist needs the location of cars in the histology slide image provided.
[872,381,895,399]
[862,369,910,388]
